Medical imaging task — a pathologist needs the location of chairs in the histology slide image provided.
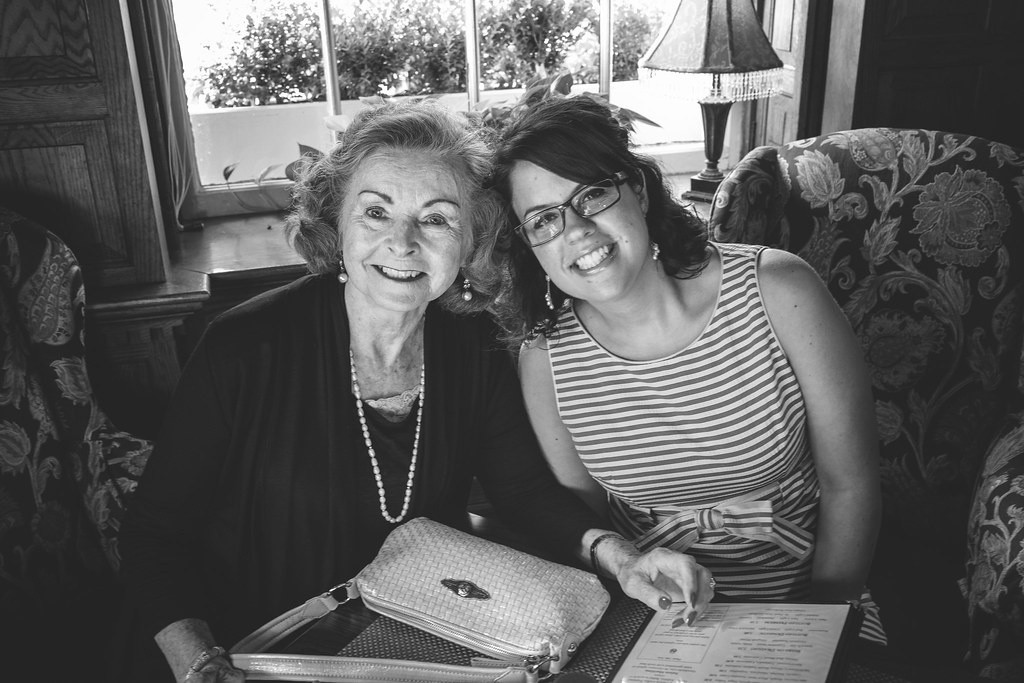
[705,126,1024,683]
[0,213,170,683]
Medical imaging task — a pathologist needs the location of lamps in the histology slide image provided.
[636,0,784,201]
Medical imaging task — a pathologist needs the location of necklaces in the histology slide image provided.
[344,339,425,525]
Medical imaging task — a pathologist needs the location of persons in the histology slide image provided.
[121,98,718,683]
[495,96,886,683]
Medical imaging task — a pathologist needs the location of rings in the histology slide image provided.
[709,577,718,589]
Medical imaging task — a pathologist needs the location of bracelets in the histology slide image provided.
[185,644,228,679]
[590,532,627,580]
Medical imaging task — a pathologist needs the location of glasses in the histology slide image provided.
[515,170,630,248]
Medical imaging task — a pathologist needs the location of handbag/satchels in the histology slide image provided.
[230,514,610,683]
